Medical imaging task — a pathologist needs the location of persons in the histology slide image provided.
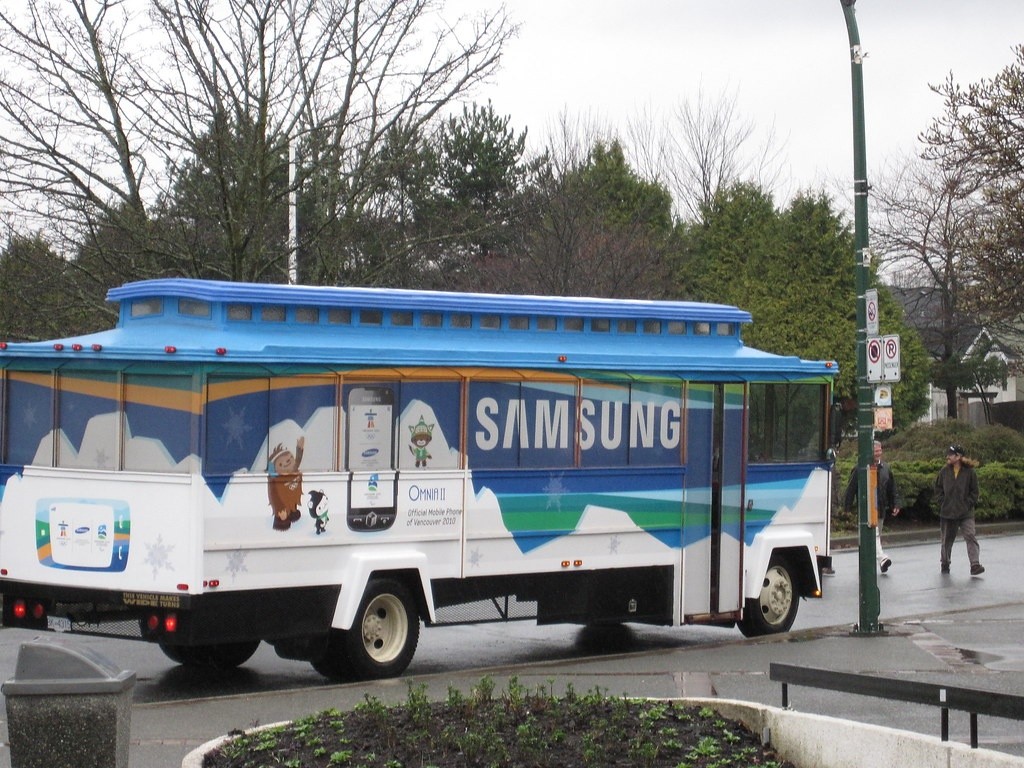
[844,440,901,572]
[934,445,984,575]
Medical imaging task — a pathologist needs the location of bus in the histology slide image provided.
[0,277,848,684]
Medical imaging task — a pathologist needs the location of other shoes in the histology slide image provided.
[880,558,891,573]
[821,568,835,574]
[941,563,950,574]
[971,564,985,575]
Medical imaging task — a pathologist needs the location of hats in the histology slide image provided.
[946,445,963,455]
